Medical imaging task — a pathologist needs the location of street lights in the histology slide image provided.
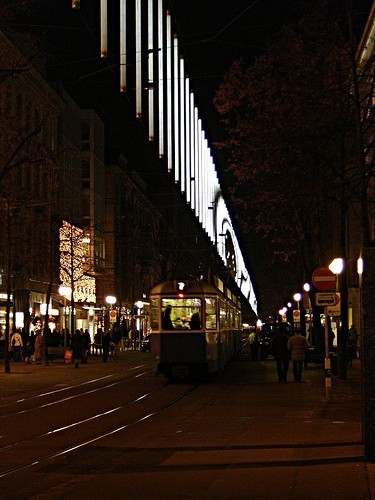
[293,292,301,310]
[135,300,144,350]
[106,295,116,362]
[58,287,72,364]
[303,283,313,345]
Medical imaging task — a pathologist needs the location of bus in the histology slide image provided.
[145,276,244,386]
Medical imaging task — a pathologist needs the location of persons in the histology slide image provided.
[8,305,201,368]
[249,324,357,383]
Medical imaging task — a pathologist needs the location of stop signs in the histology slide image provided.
[312,266,337,291]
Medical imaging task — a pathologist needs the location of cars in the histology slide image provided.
[141,335,153,352]
